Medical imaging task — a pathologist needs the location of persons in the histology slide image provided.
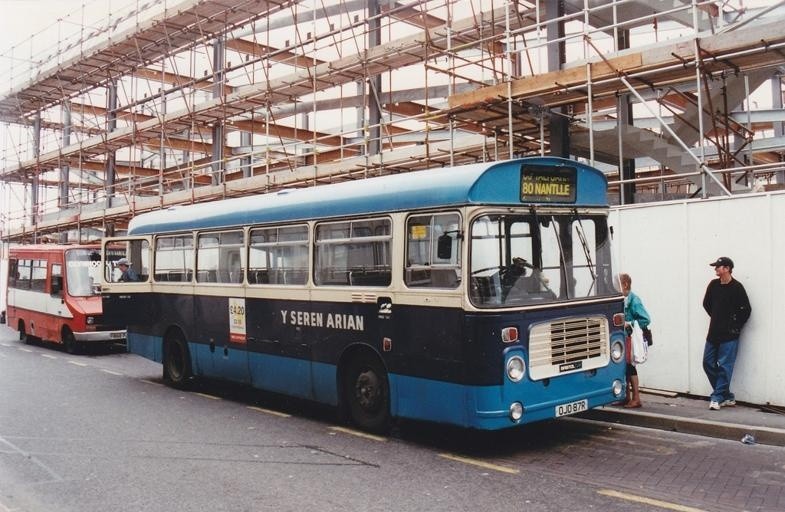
[611,275,651,408]
[114,258,141,282]
[703,258,751,411]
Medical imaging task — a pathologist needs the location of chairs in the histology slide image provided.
[155,269,191,282]
[403,265,459,289]
[248,266,308,284]
[197,268,243,283]
[313,269,391,286]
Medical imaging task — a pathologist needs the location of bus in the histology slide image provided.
[97,155,629,438]
[3,240,131,356]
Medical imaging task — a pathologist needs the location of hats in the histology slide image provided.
[708,256,734,268]
[117,257,133,267]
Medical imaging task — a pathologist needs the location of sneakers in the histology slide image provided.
[624,399,642,409]
[719,398,736,408]
[611,397,631,406]
[707,399,720,410]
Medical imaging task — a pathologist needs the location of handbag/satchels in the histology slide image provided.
[626,319,653,366]
